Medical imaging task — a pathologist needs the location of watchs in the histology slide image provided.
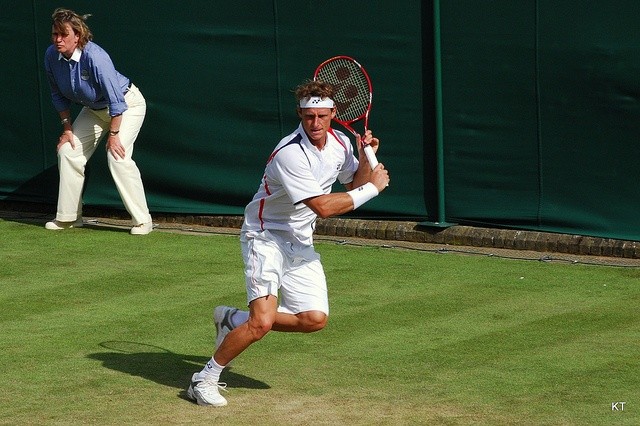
[108,129,119,136]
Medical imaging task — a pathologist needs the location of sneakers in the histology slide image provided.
[213,306,239,352]
[130,221,153,235]
[187,371,230,408]
[45,218,84,229]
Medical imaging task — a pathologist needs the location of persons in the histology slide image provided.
[187,82,390,409]
[44,9,153,237]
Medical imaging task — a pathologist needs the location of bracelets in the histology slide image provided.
[347,181,381,210]
[61,116,72,123]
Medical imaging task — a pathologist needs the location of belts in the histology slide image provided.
[91,78,132,111]
